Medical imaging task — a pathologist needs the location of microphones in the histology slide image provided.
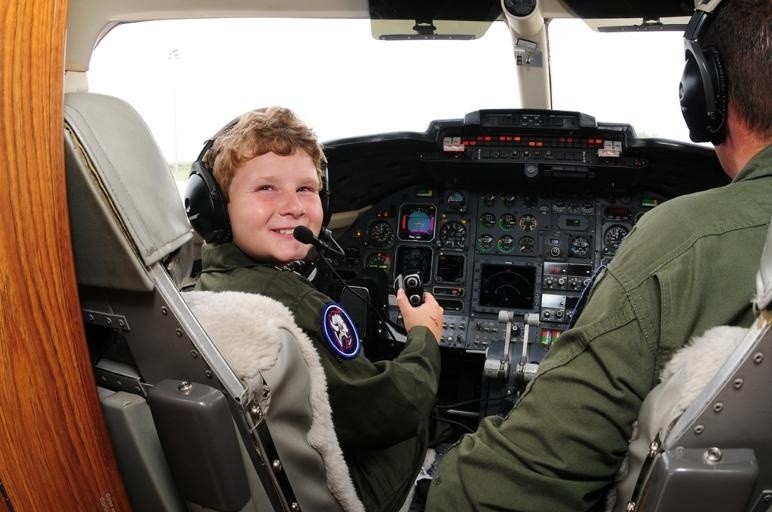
[293,225,346,258]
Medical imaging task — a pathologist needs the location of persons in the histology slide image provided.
[426,0,770,511]
[194,106,444,512]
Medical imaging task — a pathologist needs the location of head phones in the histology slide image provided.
[184,107,332,245]
[679,0,728,146]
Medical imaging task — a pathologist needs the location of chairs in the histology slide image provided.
[605,223,772,512]
[61,93,437,512]
